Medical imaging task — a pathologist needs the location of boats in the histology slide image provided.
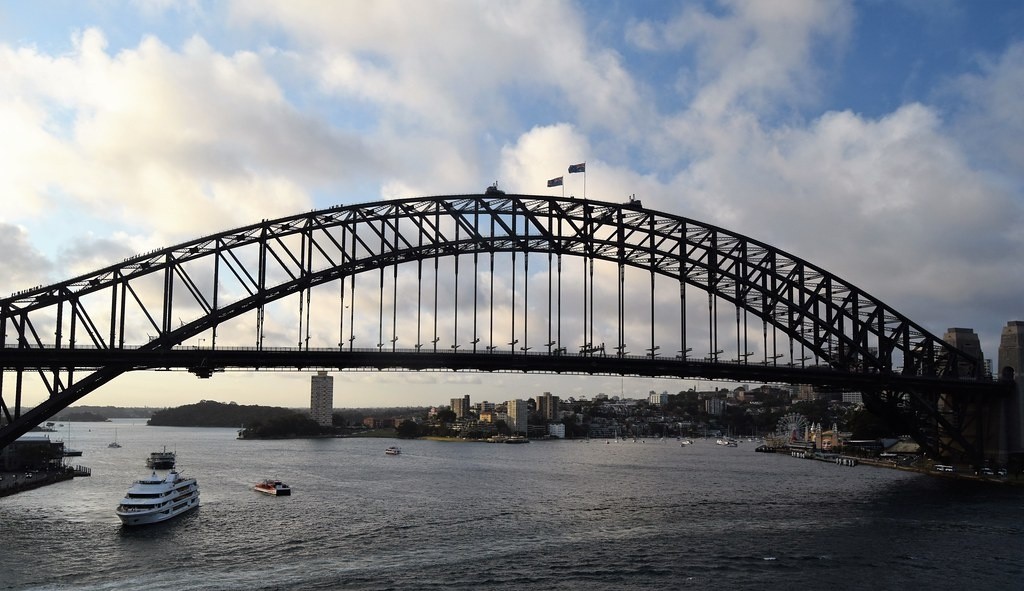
[47,422,54,425]
[253,479,291,496]
[115,469,202,527]
[385,446,401,455]
[147,451,176,470]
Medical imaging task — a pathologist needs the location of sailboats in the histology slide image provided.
[108,429,121,448]
[579,428,766,447]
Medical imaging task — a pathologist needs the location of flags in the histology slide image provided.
[547,177,563,188]
[568,163,586,173]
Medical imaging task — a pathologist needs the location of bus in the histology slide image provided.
[934,465,955,472]
[981,468,1007,475]
